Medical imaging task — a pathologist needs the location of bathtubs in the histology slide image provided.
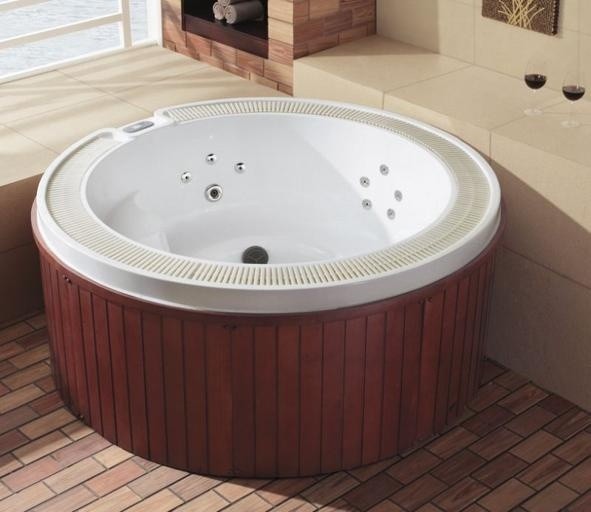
[35,97,503,317]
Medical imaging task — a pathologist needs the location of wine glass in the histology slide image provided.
[559,68,586,129]
[522,56,549,117]
